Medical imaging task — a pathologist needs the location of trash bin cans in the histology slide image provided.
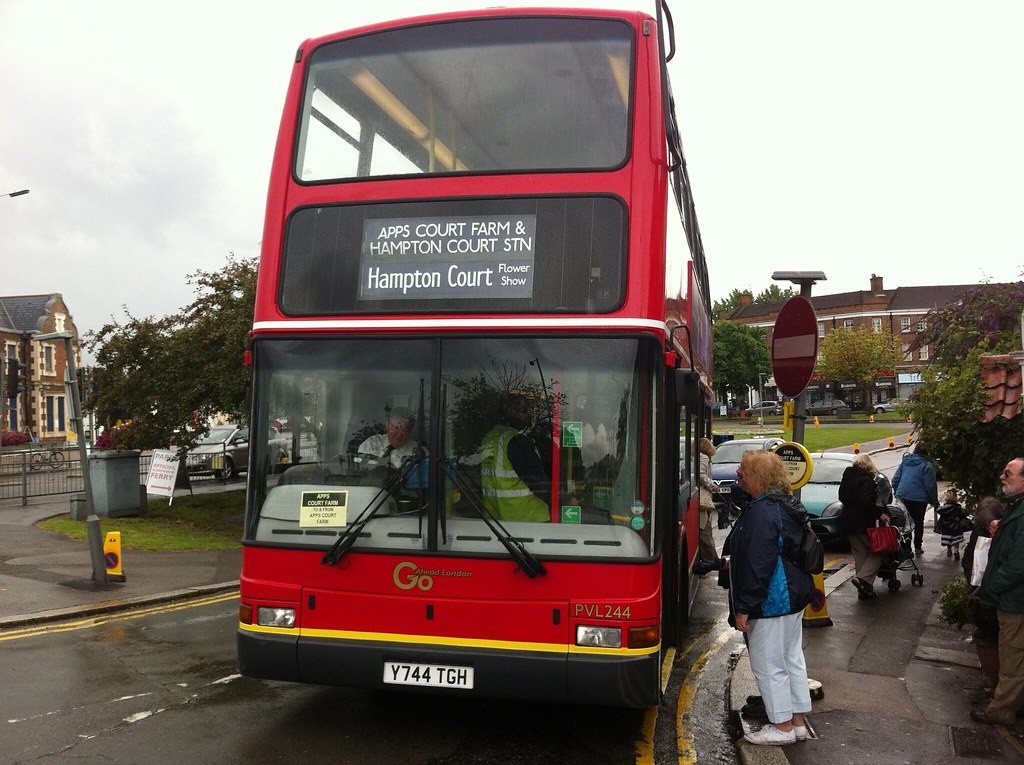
[712,434,734,447]
[88,450,141,516]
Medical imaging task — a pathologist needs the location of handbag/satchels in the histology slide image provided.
[869,517,898,553]
[699,488,715,512]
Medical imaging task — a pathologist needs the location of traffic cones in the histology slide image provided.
[100,531,126,582]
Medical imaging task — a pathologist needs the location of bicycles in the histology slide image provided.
[31,444,65,471]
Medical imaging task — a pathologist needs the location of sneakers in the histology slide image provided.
[793,726,808,741]
[743,724,797,746]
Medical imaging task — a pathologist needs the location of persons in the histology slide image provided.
[935,492,965,556]
[481,393,579,521]
[893,442,940,554]
[697,448,824,745]
[970,457,1024,723]
[354,408,430,468]
[838,455,891,597]
[961,497,1003,695]
[700,438,720,561]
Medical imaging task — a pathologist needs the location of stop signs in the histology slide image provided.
[771,295,820,399]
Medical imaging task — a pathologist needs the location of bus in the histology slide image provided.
[235,1,716,712]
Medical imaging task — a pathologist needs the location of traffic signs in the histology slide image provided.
[144,448,180,497]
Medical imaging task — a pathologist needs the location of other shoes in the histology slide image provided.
[947,550,952,555]
[955,553,959,560]
[851,576,869,598]
[858,589,875,599]
[746,695,765,703]
[742,704,767,717]
[972,710,1001,724]
[915,548,924,554]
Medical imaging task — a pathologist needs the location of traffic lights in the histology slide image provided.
[8,358,27,397]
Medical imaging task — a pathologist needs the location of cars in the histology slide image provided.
[799,452,859,535]
[181,417,322,480]
[873,398,911,414]
[707,437,787,502]
[745,399,850,417]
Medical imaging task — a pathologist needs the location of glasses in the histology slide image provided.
[1002,472,1022,477]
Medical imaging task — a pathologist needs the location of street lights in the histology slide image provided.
[32,329,108,586]
[770,269,827,441]
[304,392,318,437]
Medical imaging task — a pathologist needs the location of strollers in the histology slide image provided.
[876,502,924,590]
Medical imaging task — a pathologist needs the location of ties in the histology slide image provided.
[380,443,397,460]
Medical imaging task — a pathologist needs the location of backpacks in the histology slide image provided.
[774,500,825,574]
